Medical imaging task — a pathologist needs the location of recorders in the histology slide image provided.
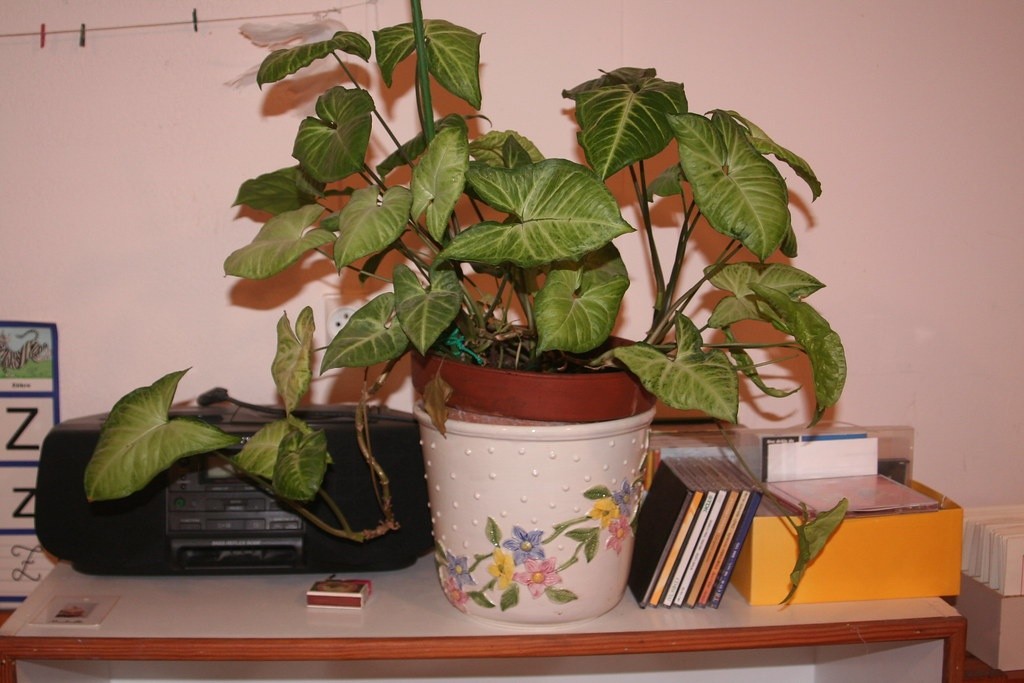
[34,402,433,578]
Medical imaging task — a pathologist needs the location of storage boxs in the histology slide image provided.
[954,572,1024,672]
[727,473,962,602]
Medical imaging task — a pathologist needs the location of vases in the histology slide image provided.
[412,405,657,625]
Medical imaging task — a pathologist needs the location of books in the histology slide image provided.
[627,457,764,610]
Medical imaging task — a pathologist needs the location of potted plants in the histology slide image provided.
[83,0,850,607]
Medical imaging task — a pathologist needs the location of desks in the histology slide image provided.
[0,551,966,683]
